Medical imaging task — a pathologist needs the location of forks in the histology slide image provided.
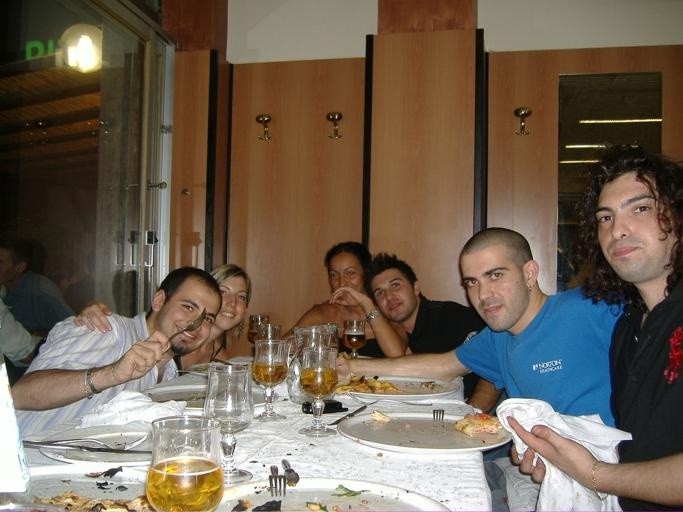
[432,407,445,421]
[47,435,147,450]
[170,307,210,341]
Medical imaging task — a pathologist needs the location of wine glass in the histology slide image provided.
[147,417,224,512]
[241,313,341,438]
[340,319,367,358]
[202,364,253,485]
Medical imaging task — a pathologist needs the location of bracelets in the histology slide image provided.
[589,456,613,503]
[89,366,101,394]
[367,308,380,322]
[84,368,95,399]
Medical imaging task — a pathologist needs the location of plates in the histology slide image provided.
[143,385,280,412]
[0,463,149,512]
[185,362,249,377]
[351,375,460,401]
[337,412,512,453]
[40,424,153,466]
[216,477,453,511]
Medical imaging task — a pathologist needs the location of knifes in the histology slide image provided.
[22,440,152,454]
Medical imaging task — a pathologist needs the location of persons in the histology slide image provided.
[281,242,409,359]
[365,250,483,403]
[11,266,221,439]
[0,297,48,368]
[502,147,682,511]
[1,241,78,332]
[333,228,635,441]
[74,265,252,378]
